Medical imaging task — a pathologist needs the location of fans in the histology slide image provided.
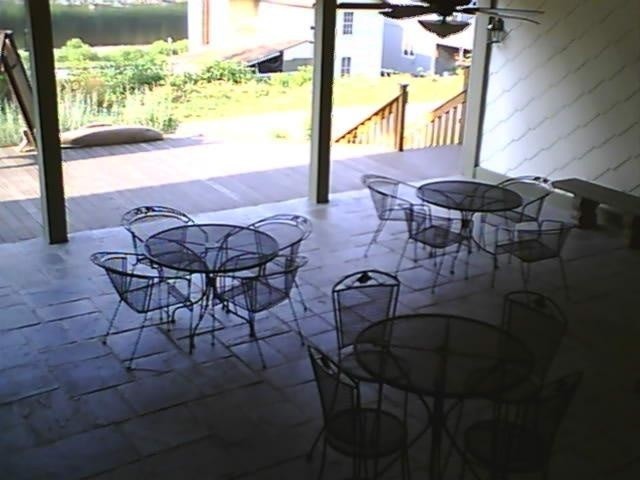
[335,0,544,39]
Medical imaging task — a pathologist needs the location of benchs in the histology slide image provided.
[552,177,640,247]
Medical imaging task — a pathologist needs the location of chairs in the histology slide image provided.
[88,205,307,371]
[361,174,574,297]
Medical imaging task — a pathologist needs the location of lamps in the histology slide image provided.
[486,15,506,42]
